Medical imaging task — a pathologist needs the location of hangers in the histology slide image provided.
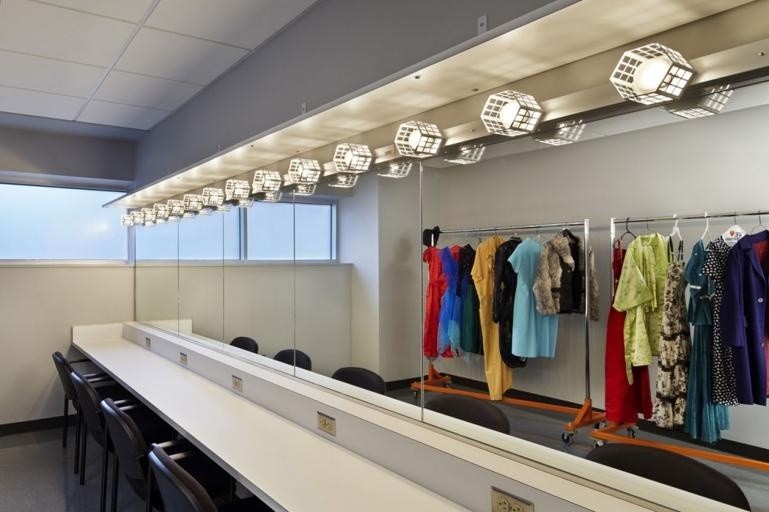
[721,213,748,244]
[665,213,683,244]
[749,211,766,237]
[640,216,658,246]
[613,217,636,252]
[432,222,579,251]
[702,210,715,247]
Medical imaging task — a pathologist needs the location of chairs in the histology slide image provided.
[51,349,262,510]
[587,440,754,511]
[424,394,512,436]
[228,337,259,354]
[274,350,313,371]
[331,365,387,394]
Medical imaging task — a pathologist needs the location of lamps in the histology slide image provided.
[331,142,372,174]
[291,186,314,197]
[120,178,250,229]
[374,160,412,179]
[656,85,733,119]
[442,146,483,166]
[392,119,441,156]
[609,42,690,109]
[261,193,281,202]
[327,176,357,190]
[288,158,321,189]
[480,90,542,139]
[532,119,585,147]
[251,167,281,193]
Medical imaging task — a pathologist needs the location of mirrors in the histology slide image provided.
[132,72,768,512]
